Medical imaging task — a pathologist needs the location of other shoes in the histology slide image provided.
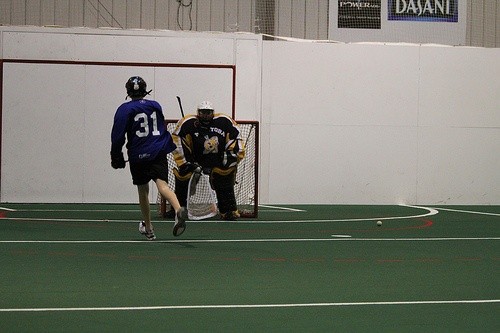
[172,207,188,237]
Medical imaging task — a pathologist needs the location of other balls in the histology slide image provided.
[377,220,382,226]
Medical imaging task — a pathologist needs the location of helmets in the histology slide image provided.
[197,100,214,116]
[126,76,147,98]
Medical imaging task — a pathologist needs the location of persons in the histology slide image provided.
[111,76,187,240]
[167,102,245,221]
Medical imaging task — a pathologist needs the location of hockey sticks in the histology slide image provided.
[176,96,218,220]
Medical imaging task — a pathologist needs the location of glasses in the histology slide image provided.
[199,110,213,116]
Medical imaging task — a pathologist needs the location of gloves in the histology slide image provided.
[111,152,125,169]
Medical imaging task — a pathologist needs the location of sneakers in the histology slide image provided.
[138,220,157,241]
